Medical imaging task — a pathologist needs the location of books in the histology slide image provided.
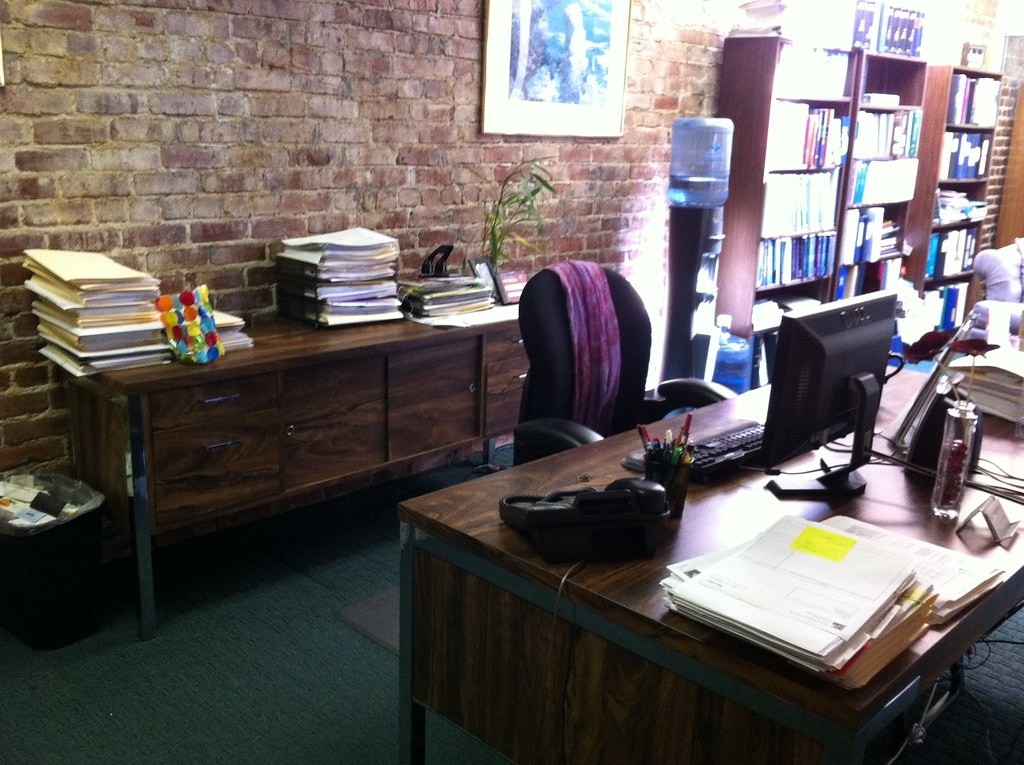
[763,171,835,237]
[405,273,497,318]
[853,160,918,205]
[274,228,405,329]
[925,185,988,281]
[843,207,900,265]
[751,291,822,333]
[923,282,970,334]
[948,75,1000,131]
[20,244,172,379]
[835,259,901,301]
[770,100,849,171]
[755,231,835,288]
[940,133,990,180]
[951,344,1024,422]
[211,307,254,349]
[853,112,920,160]
[661,518,1006,694]
[846,1,925,59]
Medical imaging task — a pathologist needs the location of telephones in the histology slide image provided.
[499,477,671,564]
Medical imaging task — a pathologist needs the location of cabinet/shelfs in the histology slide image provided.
[65,303,527,640]
[831,50,928,303]
[723,34,858,340]
[901,62,1003,300]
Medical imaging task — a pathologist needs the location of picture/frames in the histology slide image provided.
[480,0,629,137]
[468,258,501,304]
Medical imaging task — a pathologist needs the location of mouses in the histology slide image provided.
[620,451,647,472]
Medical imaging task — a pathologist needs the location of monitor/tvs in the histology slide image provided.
[757,290,897,501]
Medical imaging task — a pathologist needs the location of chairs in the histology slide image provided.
[512,260,736,462]
[973,238,1024,304]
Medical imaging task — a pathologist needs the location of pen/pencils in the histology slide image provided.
[637,413,694,519]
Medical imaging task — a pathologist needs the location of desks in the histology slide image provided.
[398,365,1024,765]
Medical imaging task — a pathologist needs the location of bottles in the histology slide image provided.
[668,117,735,206]
[711,327,753,395]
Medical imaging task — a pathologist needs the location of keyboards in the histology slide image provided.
[686,424,765,478]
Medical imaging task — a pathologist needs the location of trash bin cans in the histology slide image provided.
[0,469,118,652]
